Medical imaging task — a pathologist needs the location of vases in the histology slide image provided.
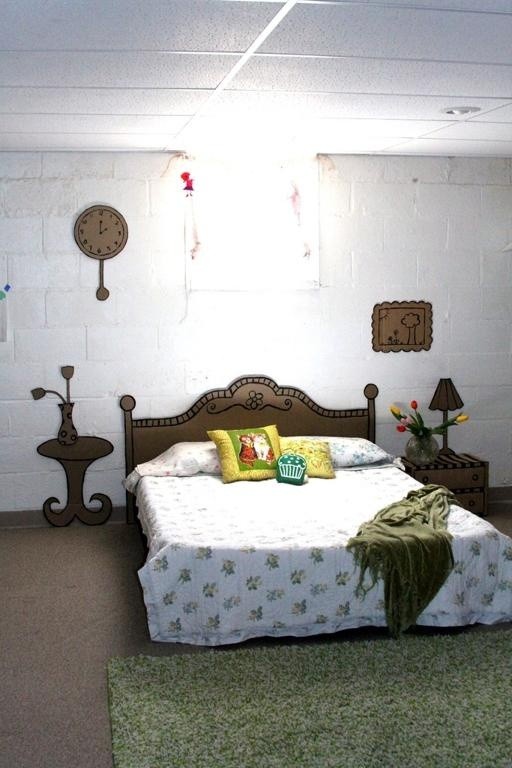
[405,436,439,466]
[57,402,79,444]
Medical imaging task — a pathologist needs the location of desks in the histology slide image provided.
[36,436,115,527]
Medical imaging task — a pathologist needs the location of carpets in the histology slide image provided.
[105,628,512,767]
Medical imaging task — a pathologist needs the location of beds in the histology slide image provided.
[122,458,512,645]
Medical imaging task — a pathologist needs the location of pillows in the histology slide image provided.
[276,454,309,487]
[278,438,336,479]
[206,424,282,483]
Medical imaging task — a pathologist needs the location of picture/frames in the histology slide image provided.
[371,299,433,353]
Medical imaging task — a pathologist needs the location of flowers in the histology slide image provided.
[388,399,469,439]
[30,365,75,406]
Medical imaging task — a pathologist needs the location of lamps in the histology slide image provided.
[428,378,465,456]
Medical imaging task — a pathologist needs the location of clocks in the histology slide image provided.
[74,205,129,301]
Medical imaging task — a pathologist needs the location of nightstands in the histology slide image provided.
[397,452,489,517]
